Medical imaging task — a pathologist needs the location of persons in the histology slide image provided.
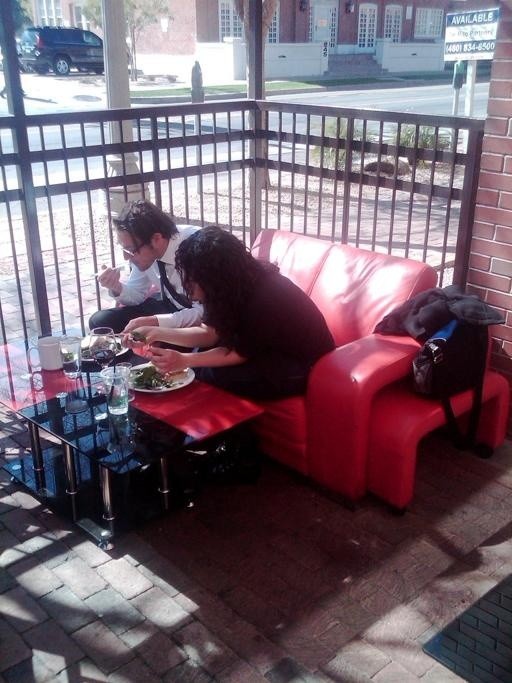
[0,45,28,100]
[121,224,336,402]
[88,199,205,333]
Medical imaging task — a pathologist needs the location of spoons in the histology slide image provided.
[129,328,150,349]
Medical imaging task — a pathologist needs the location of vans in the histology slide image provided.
[20,23,131,74]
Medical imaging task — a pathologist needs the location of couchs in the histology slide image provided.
[119,228,508,514]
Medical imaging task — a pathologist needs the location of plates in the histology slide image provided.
[81,335,130,361]
[129,361,196,394]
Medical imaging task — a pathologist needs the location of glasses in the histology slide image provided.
[113,233,153,257]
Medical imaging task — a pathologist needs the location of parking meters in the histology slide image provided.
[190,60,206,195]
[449,60,464,149]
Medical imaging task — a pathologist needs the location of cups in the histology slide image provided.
[101,366,130,415]
[65,376,90,415]
[116,362,133,401]
[109,414,131,447]
[29,335,63,370]
[57,333,82,378]
[30,368,66,394]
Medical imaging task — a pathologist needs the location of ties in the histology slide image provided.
[157,260,192,308]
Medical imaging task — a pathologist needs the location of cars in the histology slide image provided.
[0,37,33,74]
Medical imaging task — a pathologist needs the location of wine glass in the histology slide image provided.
[89,326,116,369]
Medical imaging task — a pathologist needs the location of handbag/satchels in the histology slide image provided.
[412,319,487,399]
